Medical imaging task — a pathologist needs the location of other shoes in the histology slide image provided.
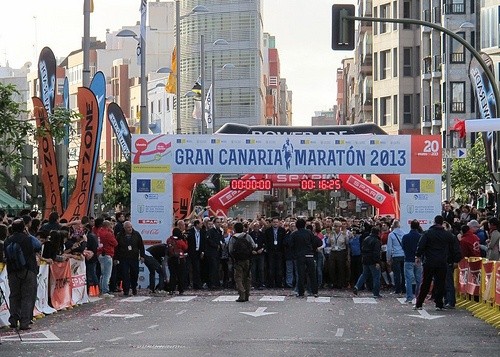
[19,324,32,331]
[445,304,456,309]
[296,294,304,298]
[131,285,137,295]
[353,286,358,295]
[124,289,130,295]
[33,313,45,321]
[310,292,318,296]
[373,293,382,298]
[405,298,413,302]
[10,323,19,328]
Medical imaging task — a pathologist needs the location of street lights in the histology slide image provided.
[157,4,210,134]
[117,30,165,134]
[185,39,235,135]
[444,22,475,201]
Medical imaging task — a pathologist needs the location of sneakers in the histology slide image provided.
[412,304,423,310]
[435,307,440,310]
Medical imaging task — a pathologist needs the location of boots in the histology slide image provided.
[244,290,250,301]
[236,291,246,302]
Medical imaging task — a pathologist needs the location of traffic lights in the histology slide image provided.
[24,175,37,199]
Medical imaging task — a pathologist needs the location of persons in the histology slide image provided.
[0,187,500,325]
[2,218,42,330]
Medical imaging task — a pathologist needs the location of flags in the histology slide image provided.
[164,44,178,95]
[453,120,466,141]
[204,83,212,128]
[184,73,202,120]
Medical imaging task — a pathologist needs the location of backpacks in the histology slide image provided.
[232,234,253,261]
[6,233,30,267]
